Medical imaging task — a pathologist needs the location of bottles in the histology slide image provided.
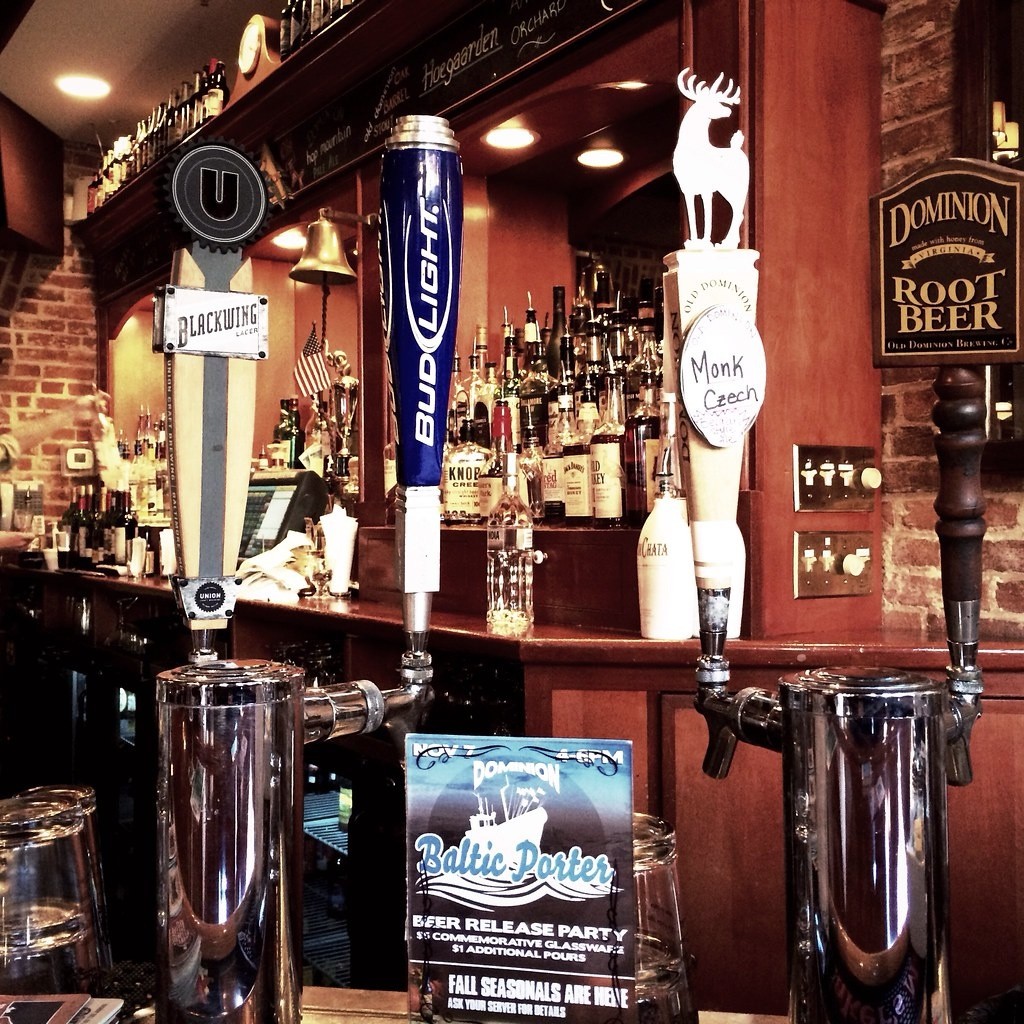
[434,655,524,735]
[274,639,344,687]
[257,394,303,471]
[0,382,176,579]
[439,249,746,641]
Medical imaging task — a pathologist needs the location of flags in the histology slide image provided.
[292,327,332,397]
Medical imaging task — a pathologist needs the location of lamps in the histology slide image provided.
[579,130,626,168]
[481,112,543,151]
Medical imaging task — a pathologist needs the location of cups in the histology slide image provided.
[0,782,118,997]
[43,548,58,570]
[319,505,360,595]
[31,515,45,535]
[144,551,154,573]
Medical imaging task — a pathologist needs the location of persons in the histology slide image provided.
[0,391,111,474]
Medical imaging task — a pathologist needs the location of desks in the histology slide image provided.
[129,983,789,1024]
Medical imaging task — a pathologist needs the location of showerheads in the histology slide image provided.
[693,685,784,781]
[303,653,437,772]
[941,663,985,788]
